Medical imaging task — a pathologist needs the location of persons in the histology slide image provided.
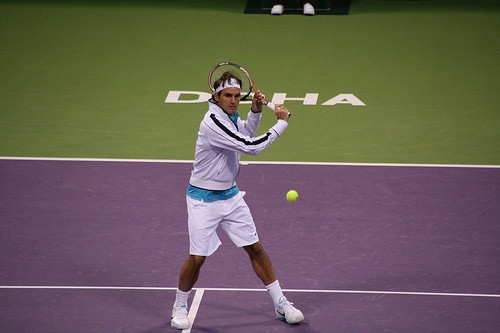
[271,0,315,16]
[170,72,304,330]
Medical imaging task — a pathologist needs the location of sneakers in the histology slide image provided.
[275,296,304,324]
[171,304,190,329]
[271,5,283,14]
[304,3,315,14]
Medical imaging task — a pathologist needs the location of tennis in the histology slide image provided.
[285,189,299,203]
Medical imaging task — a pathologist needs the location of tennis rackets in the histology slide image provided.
[207,61,293,120]
